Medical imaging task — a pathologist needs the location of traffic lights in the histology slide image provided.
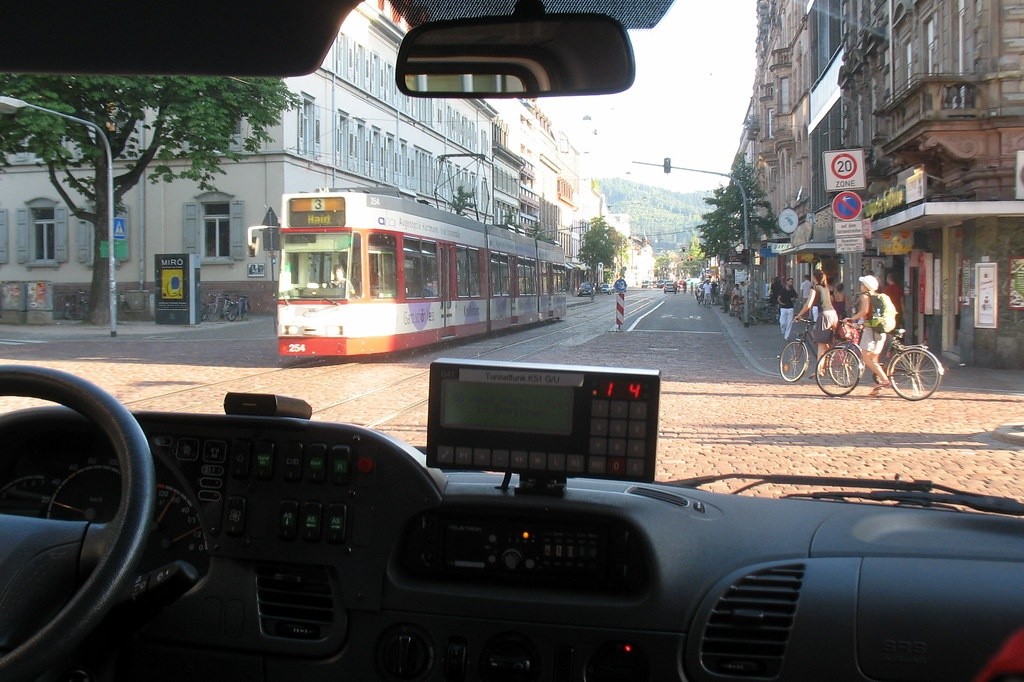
[664,158,671,173]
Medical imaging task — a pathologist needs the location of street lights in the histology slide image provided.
[0,95,117,338]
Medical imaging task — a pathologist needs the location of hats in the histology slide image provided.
[858,274,879,291]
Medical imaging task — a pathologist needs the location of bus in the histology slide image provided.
[246,189,567,361]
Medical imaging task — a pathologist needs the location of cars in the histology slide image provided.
[599,283,612,293]
[640,279,704,300]
[578,282,596,297]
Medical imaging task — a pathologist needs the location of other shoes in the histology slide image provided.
[868,379,894,396]
[809,368,827,380]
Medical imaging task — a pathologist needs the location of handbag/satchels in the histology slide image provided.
[835,320,859,340]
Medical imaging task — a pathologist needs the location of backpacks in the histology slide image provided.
[861,292,898,333]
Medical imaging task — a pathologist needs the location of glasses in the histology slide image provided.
[859,283,864,286]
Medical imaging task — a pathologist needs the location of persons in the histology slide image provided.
[830,283,852,320]
[843,275,892,398]
[793,270,838,378]
[777,278,799,341]
[333,264,355,295]
[674,276,783,311]
[800,275,812,321]
[880,268,906,327]
[827,275,834,299]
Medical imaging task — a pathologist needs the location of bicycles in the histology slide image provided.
[815,317,945,401]
[201,290,251,323]
[727,293,780,325]
[62,290,90,321]
[779,315,865,389]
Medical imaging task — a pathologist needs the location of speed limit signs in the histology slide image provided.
[821,147,866,193]
